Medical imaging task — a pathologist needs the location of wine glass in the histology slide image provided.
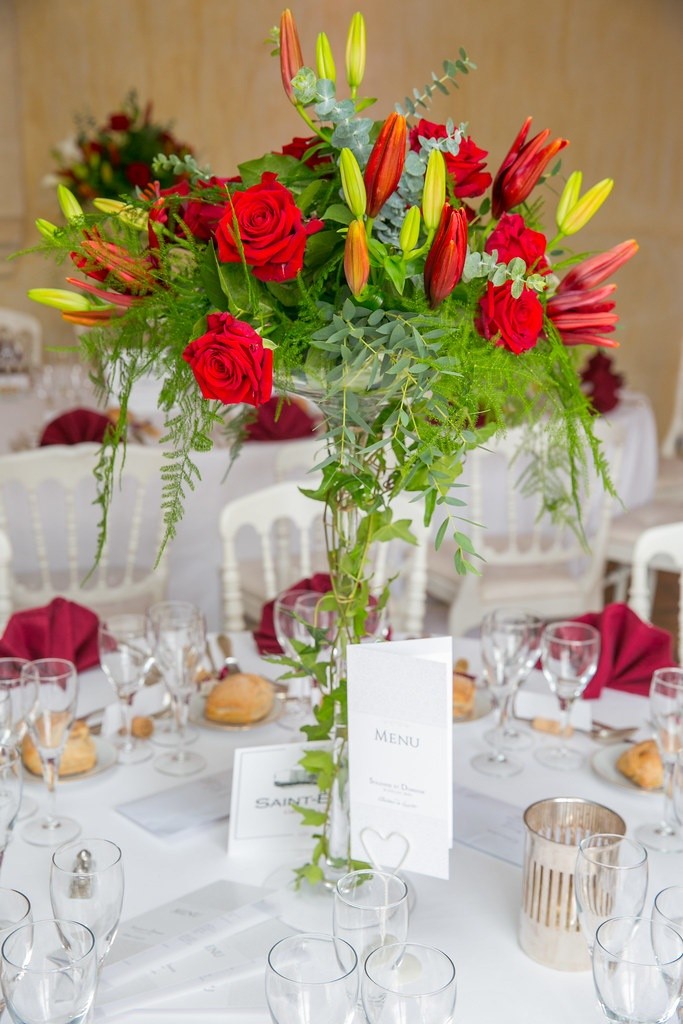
[97,601,207,777]
[470,606,600,779]
[0,658,82,870]
[332,870,408,1024]
[272,590,389,742]
[635,664,683,852]
[572,833,652,1012]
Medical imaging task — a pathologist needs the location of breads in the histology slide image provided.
[202,672,274,723]
[615,738,664,789]
[453,669,475,717]
[22,710,98,778]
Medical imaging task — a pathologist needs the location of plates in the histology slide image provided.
[592,741,674,796]
[188,694,284,732]
[11,735,111,782]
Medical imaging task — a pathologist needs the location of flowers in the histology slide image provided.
[38,94,199,197]
[27,9,648,875]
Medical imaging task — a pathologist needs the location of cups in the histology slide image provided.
[673,747,683,823]
[265,932,357,1024]
[591,884,682,1022]
[518,797,627,973]
[0,837,126,1024]
[362,944,458,1024]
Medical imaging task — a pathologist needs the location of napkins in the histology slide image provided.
[253,574,381,655]
[240,397,317,443]
[585,352,621,415]
[533,604,675,701]
[37,410,121,444]
[1,595,118,678]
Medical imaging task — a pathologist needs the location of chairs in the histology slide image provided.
[608,387,683,627]
[447,416,621,636]
[221,482,430,635]
[0,447,173,636]
